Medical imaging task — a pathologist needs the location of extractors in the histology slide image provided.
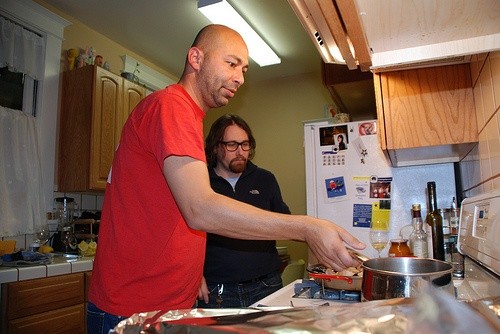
[288,0,500,85]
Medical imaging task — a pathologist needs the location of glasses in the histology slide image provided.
[217,140,253,151]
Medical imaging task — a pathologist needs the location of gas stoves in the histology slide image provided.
[291,192,500,309]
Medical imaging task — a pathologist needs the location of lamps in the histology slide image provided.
[197,1,282,68]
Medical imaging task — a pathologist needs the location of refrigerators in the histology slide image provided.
[304,119,457,279]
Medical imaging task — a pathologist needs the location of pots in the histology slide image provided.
[306,264,363,291]
[344,246,454,301]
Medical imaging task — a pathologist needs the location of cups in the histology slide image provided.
[78,245,95,257]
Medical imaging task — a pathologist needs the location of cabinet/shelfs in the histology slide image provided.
[0,270,92,334]
[320,58,478,167]
[54,67,146,194]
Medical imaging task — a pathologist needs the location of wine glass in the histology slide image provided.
[36,224,50,254]
[370,222,389,258]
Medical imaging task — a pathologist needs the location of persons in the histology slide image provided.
[86,25,366,334]
[337,135,345,150]
[196,113,291,309]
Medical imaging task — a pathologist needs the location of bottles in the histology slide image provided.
[388,239,411,257]
[409,204,428,258]
[423,182,445,262]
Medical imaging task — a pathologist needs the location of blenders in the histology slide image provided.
[51,196,80,253]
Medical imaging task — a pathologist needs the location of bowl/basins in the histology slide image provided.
[0,240,16,255]
